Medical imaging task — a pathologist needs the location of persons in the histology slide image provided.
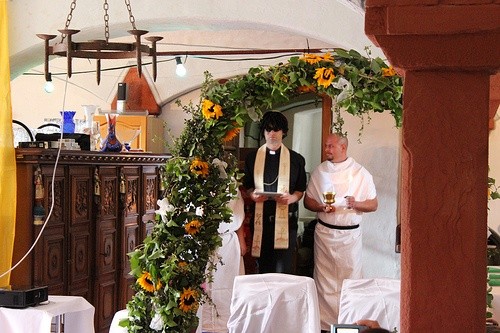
[303,133,378,333]
[240,111,307,275]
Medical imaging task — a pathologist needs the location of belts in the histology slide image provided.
[263,211,297,222]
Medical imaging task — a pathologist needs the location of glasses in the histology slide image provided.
[265,126,283,132]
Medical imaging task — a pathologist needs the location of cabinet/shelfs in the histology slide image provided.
[10,148,174,333]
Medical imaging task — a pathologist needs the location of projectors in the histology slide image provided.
[0,287,49,308]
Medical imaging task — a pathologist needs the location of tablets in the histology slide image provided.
[254,191,282,199]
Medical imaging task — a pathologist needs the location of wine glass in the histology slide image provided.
[324,185,336,210]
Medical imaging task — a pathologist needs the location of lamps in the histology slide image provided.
[36,0,163,82]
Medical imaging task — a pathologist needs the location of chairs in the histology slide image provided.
[227,273,321,333]
[337,278,401,333]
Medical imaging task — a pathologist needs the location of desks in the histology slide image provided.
[0,296,95,333]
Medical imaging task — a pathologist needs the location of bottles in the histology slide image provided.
[60,111,75,134]
[92,121,102,152]
[103,124,122,153]
[82,104,96,133]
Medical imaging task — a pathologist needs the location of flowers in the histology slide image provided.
[118,50,406,333]
